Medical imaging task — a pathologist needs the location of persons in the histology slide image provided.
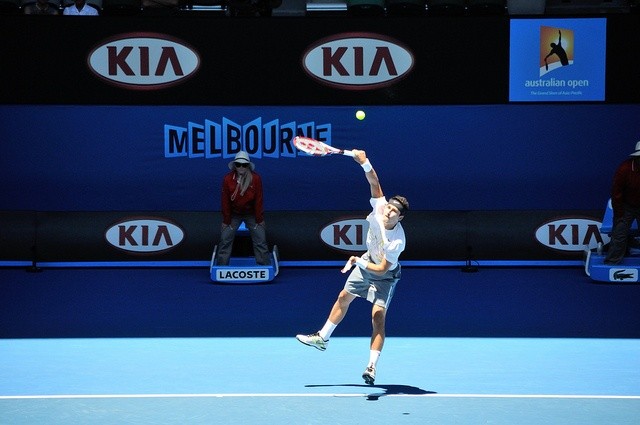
[217,151,270,266]
[296,150,410,386]
[605,128,636,265]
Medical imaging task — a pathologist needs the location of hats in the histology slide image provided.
[629,141,640,156]
[229,151,255,171]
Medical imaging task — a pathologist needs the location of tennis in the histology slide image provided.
[356,110,366,120]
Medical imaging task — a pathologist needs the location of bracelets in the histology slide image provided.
[355,258,370,271]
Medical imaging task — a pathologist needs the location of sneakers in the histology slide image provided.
[298,332,329,351]
[363,372,375,386]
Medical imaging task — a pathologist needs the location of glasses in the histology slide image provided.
[233,162,250,168]
